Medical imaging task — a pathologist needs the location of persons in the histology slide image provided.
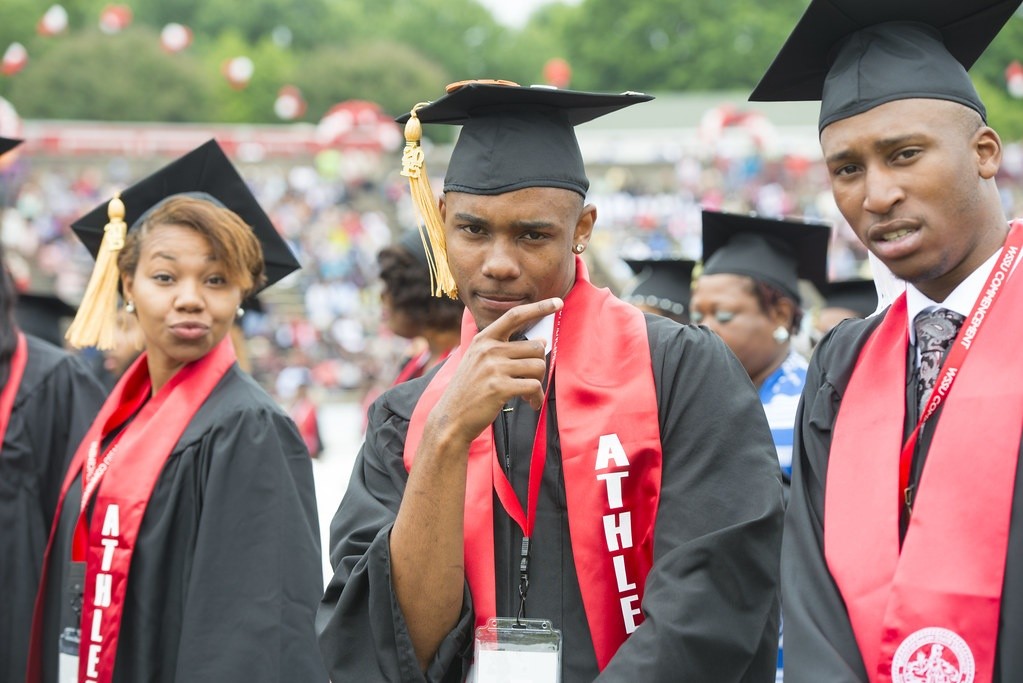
[0,97,453,463]
[0,136,110,683]
[312,79,788,683]
[744,0,1023,683]
[582,94,878,683]
[20,138,328,683]
[372,220,468,388]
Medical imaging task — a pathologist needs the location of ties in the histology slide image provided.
[913,309,964,425]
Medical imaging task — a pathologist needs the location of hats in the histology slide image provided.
[9,292,77,346]
[403,222,433,267]
[0,137,24,158]
[615,258,694,323]
[68,139,302,351]
[394,80,655,302]
[748,0,1023,143]
[698,210,832,300]
[825,278,876,317]
